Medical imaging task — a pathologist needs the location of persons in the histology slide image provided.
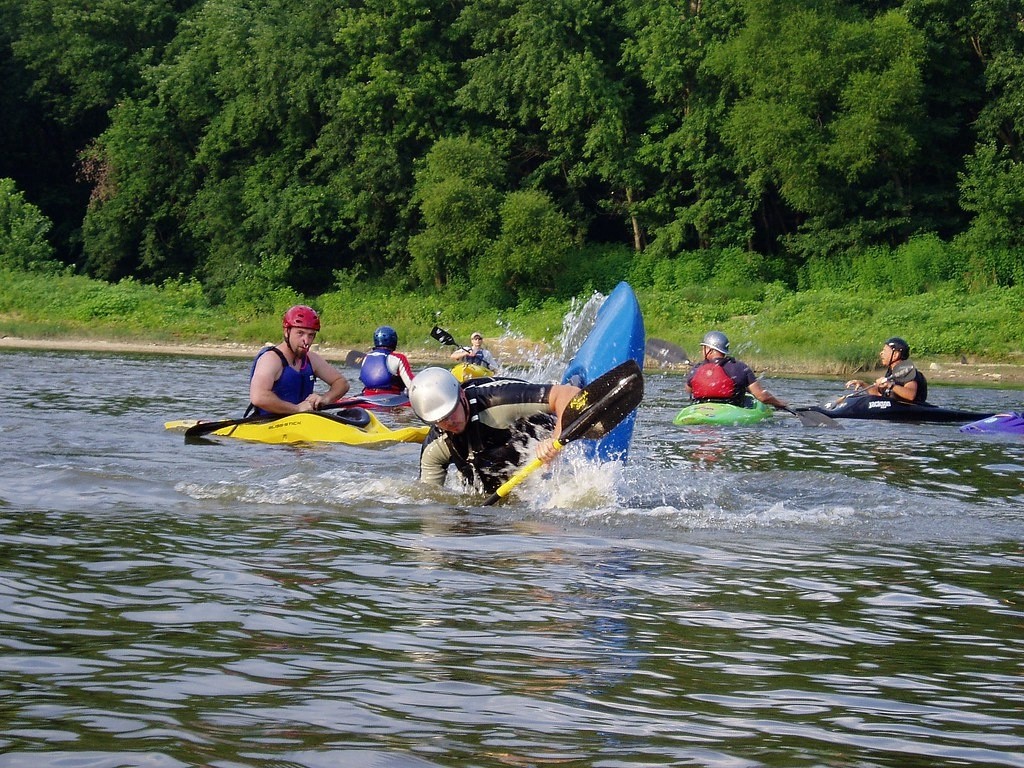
[250,305,350,415]
[410,367,581,487]
[451,333,498,375]
[686,332,788,411]
[360,326,414,389]
[846,338,921,404]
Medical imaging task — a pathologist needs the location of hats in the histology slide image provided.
[471,332,482,339]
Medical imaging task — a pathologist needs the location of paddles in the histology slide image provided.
[345,350,368,370]
[478,357,647,508]
[429,326,490,367]
[645,335,847,432]
[183,392,410,439]
[822,359,917,410]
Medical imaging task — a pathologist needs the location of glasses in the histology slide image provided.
[474,338,482,340]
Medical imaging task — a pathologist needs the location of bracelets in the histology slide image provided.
[889,383,895,390]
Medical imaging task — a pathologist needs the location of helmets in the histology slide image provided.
[409,367,460,424]
[374,326,397,347]
[700,332,730,354]
[885,337,909,360]
[283,306,320,332]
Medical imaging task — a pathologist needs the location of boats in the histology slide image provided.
[536,281,646,480]
[331,394,412,413]
[788,392,996,420]
[450,362,495,384]
[956,408,1023,435]
[163,405,434,446]
[670,391,774,428]
